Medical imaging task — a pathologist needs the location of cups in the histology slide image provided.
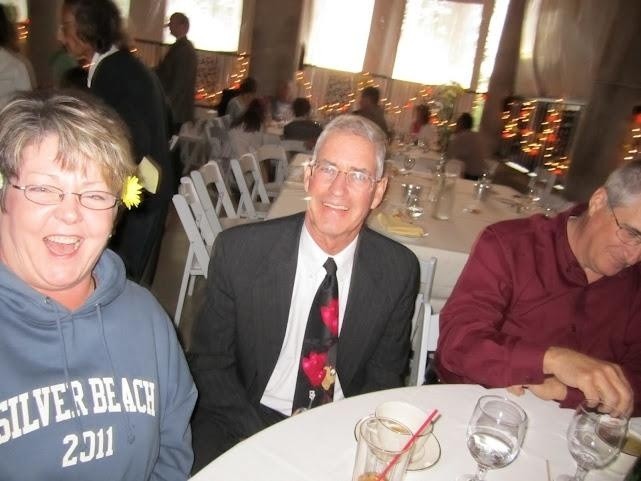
[351,416,417,481]
[406,193,426,221]
[400,183,422,207]
[474,183,492,202]
[417,137,425,150]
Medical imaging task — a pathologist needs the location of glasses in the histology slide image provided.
[315,164,375,186]
[7,178,119,210]
[608,198,641,247]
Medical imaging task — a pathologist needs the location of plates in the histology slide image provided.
[354,415,441,471]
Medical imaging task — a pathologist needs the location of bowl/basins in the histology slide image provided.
[375,400,434,458]
[595,423,641,475]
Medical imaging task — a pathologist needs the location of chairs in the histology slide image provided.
[172,107,312,328]
[412,302,442,386]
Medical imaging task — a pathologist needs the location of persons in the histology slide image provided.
[186,114,420,476]
[217,78,260,133]
[352,87,388,136]
[425,161,641,420]
[0,4,33,102]
[156,12,196,137]
[447,112,500,181]
[284,97,323,155]
[57,0,175,283]
[407,104,439,144]
[0,83,200,481]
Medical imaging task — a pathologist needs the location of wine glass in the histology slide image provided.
[459,395,529,481]
[558,399,629,481]
[404,157,415,176]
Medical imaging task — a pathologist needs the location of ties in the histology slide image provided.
[291,257,338,416]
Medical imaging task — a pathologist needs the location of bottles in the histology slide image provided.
[431,160,464,221]
